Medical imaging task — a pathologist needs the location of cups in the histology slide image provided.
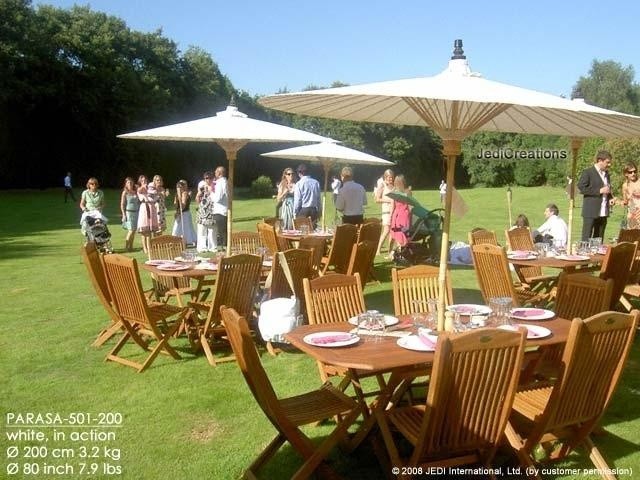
[359,310,386,334]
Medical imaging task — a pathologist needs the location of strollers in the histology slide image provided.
[392,207,449,268]
[80,206,114,256]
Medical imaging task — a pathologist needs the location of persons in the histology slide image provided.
[510,214,530,231]
[532,203,568,248]
[577,150,616,247]
[617,162,640,257]
[64,172,77,203]
[121,165,413,259]
[439,180,447,202]
[79,177,106,212]
[565,175,572,198]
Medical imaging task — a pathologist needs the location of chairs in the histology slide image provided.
[81,217,640,480]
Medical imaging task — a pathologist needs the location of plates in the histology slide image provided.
[555,254,591,261]
[396,335,439,352]
[446,304,492,316]
[348,313,399,329]
[145,253,276,273]
[496,324,552,340]
[508,307,555,320]
[304,331,360,348]
[508,255,537,259]
[598,249,608,255]
[277,225,335,236]
[506,251,538,256]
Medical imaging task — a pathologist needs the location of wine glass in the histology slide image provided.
[489,298,513,328]
[412,298,439,332]
[539,237,602,259]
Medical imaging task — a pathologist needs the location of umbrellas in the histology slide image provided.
[116,94,341,256]
[260,140,396,235]
[566,96,584,256]
[257,39,640,331]
